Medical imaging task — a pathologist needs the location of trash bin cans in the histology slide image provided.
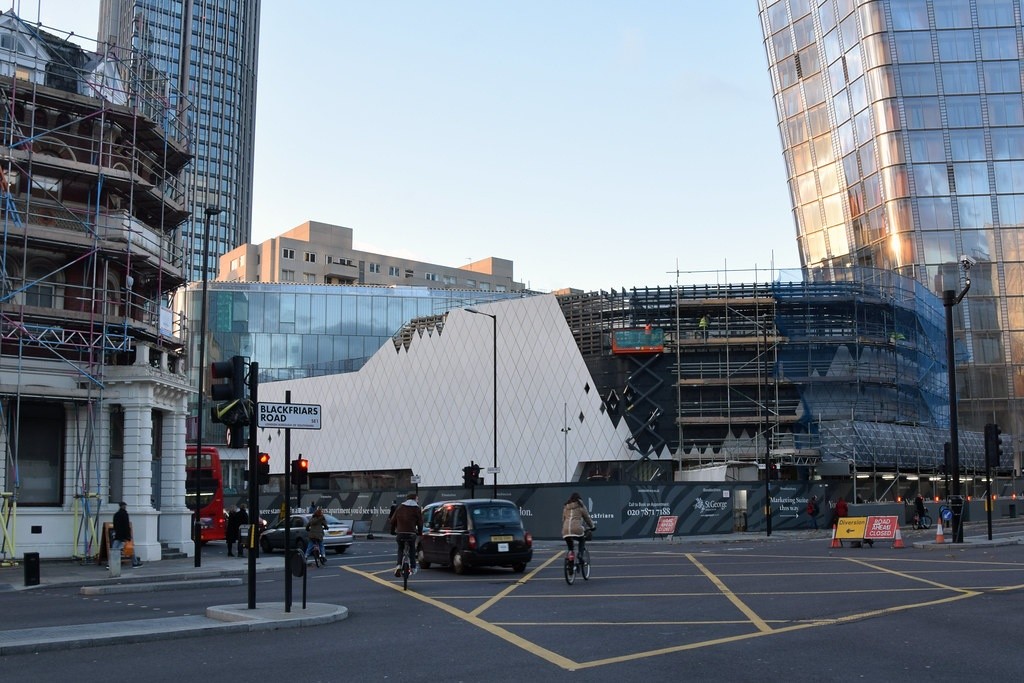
[24,552,40,586]
[1009,504,1016,517]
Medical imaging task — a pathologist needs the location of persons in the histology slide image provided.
[915,493,929,529]
[809,495,819,530]
[856,493,863,504]
[305,510,329,562]
[835,497,848,518]
[308,501,316,513]
[225,504,248,558]
[389,500,397,535]
[391,493,423,577]
[645,320,652,345]
[105,502,144,570]
[698,314,710,339]
[561,493,595,577]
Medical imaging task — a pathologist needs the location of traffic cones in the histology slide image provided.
[932,517,948,544]
[828,524,843,548]
[890,522,907,549]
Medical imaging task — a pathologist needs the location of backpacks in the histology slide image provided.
[807,502,815,513]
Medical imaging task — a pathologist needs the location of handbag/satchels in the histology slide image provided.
[123,540,134,556]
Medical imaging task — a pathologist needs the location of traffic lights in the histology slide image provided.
[561,403,572,482]
[257,453,270,485]
[300,460,308,484]
[462,466,470,489]
[984,424,1003,467]
[211,355,250,427]
[226,427,243,448]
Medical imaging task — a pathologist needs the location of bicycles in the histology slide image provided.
[564,527,596,585]
[305,528,329,568]
[912,507,932,530]
[390,531,422,591]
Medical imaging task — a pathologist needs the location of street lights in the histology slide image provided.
[194,207,221,566]
[463,307,497,498]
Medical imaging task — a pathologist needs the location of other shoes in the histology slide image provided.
[238,555,246,557]
[412,569,416,574]
[133,564,142,568]
[569,566,573,575]
[228,553,234,557]
[394,565,402,576]
[577,554,584,564]
[322,556,328,561]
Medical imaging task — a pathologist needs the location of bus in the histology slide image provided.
[183,446,227,546]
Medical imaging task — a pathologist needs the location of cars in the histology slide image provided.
[260,513,354,556]
[414,498,533,575]
[420,501,451,533]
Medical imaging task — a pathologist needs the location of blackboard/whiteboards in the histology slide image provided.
[100,521,133,560]
